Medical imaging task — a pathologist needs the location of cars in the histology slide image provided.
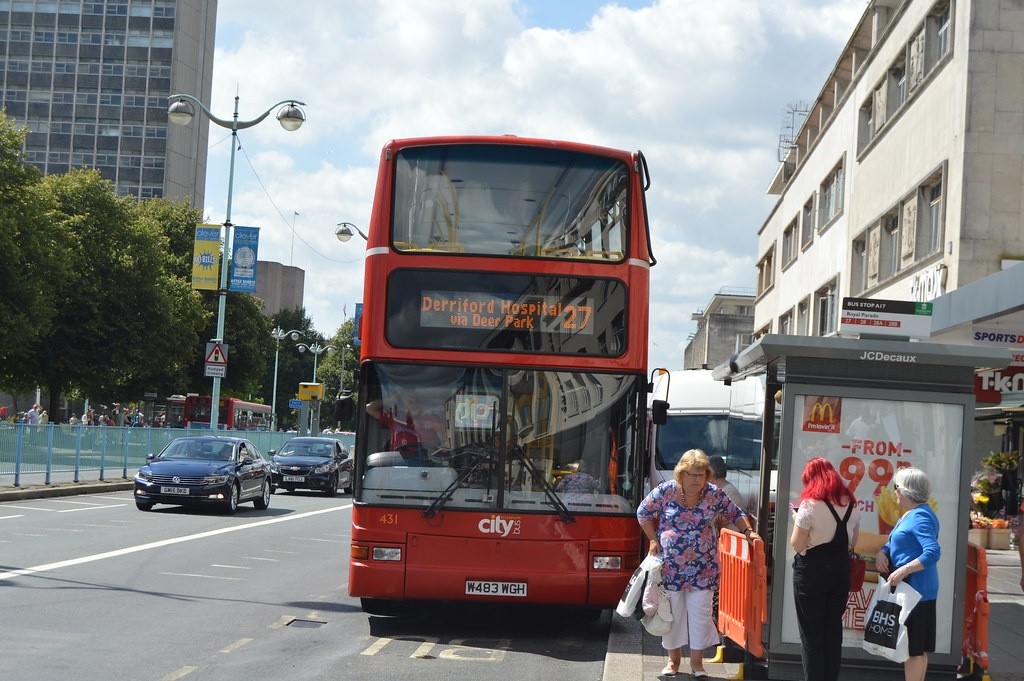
[267,437,355,497]
[133,435,274,515]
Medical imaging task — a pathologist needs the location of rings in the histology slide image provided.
[877,566,881,570]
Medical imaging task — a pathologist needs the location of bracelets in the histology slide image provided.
[743,527,753,533]
[907,562,912,574]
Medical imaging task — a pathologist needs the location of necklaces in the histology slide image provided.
[680,487,703,510]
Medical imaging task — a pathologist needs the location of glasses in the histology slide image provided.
[893,484,898,490]
[683,470,706,480]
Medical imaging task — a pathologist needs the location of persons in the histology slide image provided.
[334,426,343,433]
[366,385,452,451]
[81,403,121,447]
[322,426,332,434]
[27,402,48,445]
[128,406,166,427]
[636,447,762,679]
[115,407,133,445]
[0,403,7,419]
[219,446,232,460]
[69,413,78,432]
[790,456,862,681]
[708,454,738,646]
[875,464,941,681]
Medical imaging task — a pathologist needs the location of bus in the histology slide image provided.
[183,393,272,432]
[346,137,671,624]
[166,395,187,428]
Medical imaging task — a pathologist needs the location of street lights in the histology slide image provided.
[166,84,306,428]
[260,326,306,432]
[294,344,340,432]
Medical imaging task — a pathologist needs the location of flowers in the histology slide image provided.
[966,450,1024,528]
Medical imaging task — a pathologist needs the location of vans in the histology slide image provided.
[646,369,728,512]
[729,369,781,583]
[147,404,166,427]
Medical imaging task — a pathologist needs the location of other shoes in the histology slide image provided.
[690,666,708,680]
[662,661,678,676]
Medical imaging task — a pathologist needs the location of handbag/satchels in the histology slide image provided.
[616,553,660,618]
[635,572,649,619]
[640,586,673,637]
[863,576,922,664]
[642,557,664,617]
[881,565,911,593]
[848,547,866,592]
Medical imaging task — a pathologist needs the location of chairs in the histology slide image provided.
[396,241,625,264]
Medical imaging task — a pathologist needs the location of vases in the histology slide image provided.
[968,529,991,549]
[986,528,1015,550]
